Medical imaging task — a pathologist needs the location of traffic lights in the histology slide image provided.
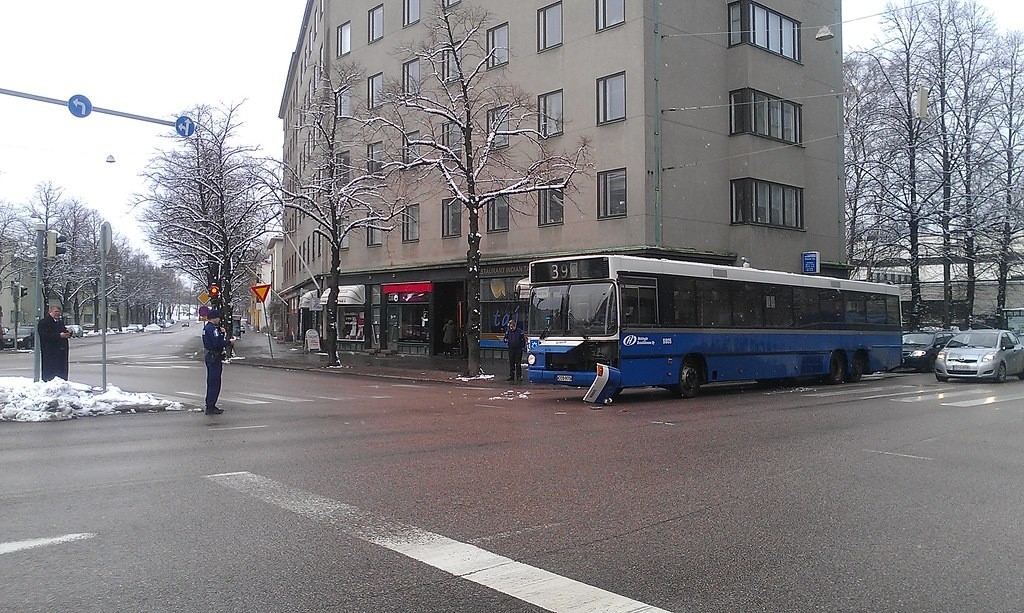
[209,285,218,307]
[46,231,67,258]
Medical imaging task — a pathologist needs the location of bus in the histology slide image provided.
[1002,308,1024,336]
[512,253,905,407]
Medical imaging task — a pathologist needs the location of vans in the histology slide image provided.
[182,321,189,327]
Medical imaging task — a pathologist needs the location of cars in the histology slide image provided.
[70,325,83,338]
[901,331,957,372]
[933,329,1024,382]
[241,325,245,333]
[2,327,35,349]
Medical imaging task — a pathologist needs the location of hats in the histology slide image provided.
[207,310,221,319]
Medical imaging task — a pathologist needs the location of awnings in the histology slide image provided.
[299,289,317,309]
[319,285,366,305]
[381,280,432,294]
[516,277,531,298]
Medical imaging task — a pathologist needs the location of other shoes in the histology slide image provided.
[506,376,511,381]
[205,406,224,415]
[517,375,522,381]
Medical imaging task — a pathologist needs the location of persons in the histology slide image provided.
[202,309,236,415]
[37,305,71,383]
[442,319,457,358]
[503,320,526,382]
